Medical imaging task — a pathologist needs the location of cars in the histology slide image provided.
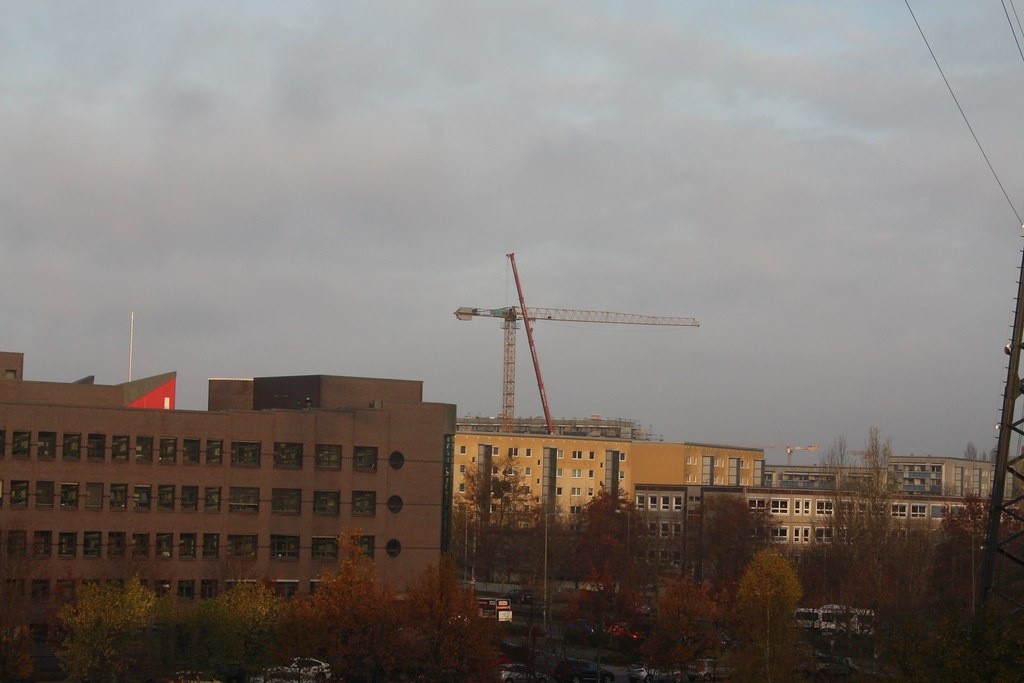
[565,619,603,646]
[171,655,332,683]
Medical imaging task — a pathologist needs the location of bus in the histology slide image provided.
[794,606,875,634]
[476,597,513,623]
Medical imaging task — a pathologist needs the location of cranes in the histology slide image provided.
[506,251,556,435]
[768,441,821,466]
[455,302,702,433]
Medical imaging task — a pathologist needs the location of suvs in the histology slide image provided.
[555,659,616,683]
[628,661,682,683]
[687,658,734,683]
